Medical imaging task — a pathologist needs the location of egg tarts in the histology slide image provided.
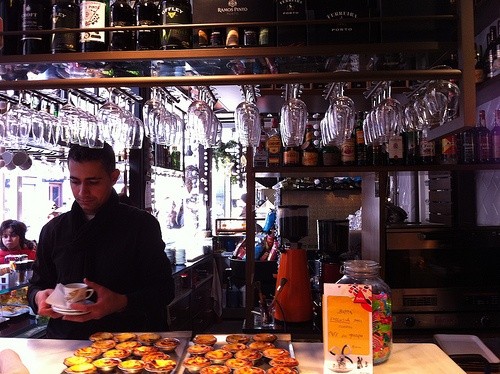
[63,332,180,374]
[183,333,299,374]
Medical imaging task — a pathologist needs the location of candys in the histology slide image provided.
[372,292,392,362]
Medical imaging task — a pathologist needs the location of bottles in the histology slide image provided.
[0,0,162,56]
[334,260,392,364]
[197,27,271,47]
[461,108,500,163]
[383,134,408,165]
[353,118,371,167]
[322,147,339,165]
[267,118,282,168]
[339,134,356,166]
[281,145,301,167]
[368,142,383,166]
[253,119,268,168]
[467,19,500,91]
[160,0,192,51]
[416,130,436,164]
[302,125,320,166]
[441,130,461,164]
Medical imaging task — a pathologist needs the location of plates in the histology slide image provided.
[49,300,94,315]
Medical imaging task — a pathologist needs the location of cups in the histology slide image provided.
[64,283,95,310]
[0,150,33,170]
[165,248,186,268]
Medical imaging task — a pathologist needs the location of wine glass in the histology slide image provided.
[319,69,461,148]
[0,85,143,150]
[143,83,307,146]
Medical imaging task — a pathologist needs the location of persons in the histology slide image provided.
[0,220,38,264]
[25,140,175,341]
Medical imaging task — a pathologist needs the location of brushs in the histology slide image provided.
[268,278,287,316]
[255,281,270,323]
